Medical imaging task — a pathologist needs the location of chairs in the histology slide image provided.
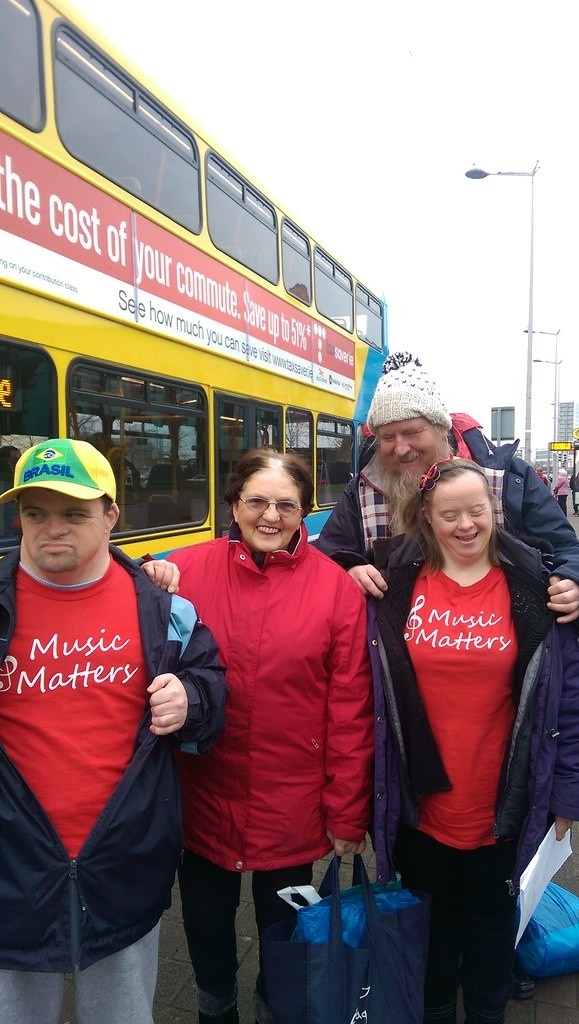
[333,318,347,329]
[318,458,352,503]
[117,175,142,194]
[121,455,241,529]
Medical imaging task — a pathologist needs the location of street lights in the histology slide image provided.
[524,328,561,488]
[464,159,541,464]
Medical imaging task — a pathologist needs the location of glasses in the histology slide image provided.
[237,495,303,518]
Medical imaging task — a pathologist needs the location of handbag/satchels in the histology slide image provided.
[260,850,430,1024]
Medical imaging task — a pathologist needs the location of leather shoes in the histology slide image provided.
[510,964,538,1001]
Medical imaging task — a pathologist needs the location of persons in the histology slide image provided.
[363,454,578,1024]
[569,472,579,516]
[536,467,549,487]
[314,353,579,1004]
[134,447,375,1024]
[552,469,570,518]
[0,438,230,1024]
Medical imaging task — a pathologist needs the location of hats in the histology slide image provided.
[0,438,117,504]
[366,351,452,437]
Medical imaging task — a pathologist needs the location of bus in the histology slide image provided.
[0,1,390,570]
